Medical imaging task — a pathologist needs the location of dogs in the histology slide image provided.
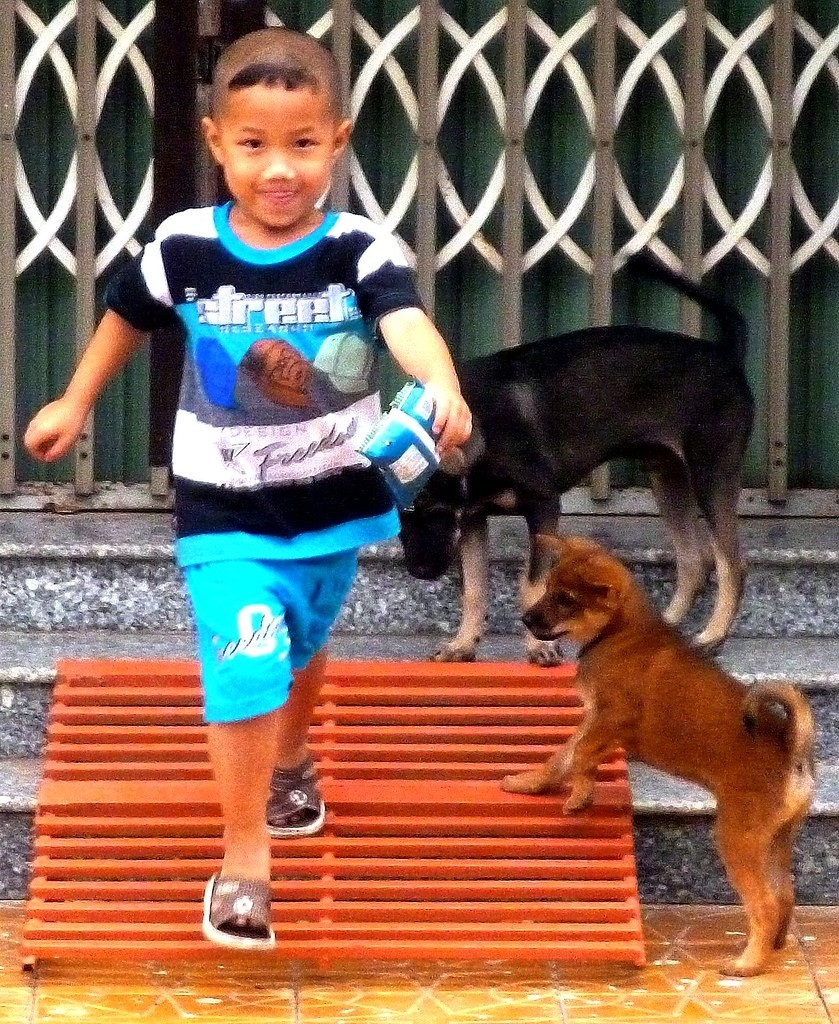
[501,534,824,979]
[395,246,760,660]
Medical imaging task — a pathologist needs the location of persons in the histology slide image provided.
[24,29,473,949]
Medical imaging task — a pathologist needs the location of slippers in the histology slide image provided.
[203,872,275,950]
[265,754,325,839]
